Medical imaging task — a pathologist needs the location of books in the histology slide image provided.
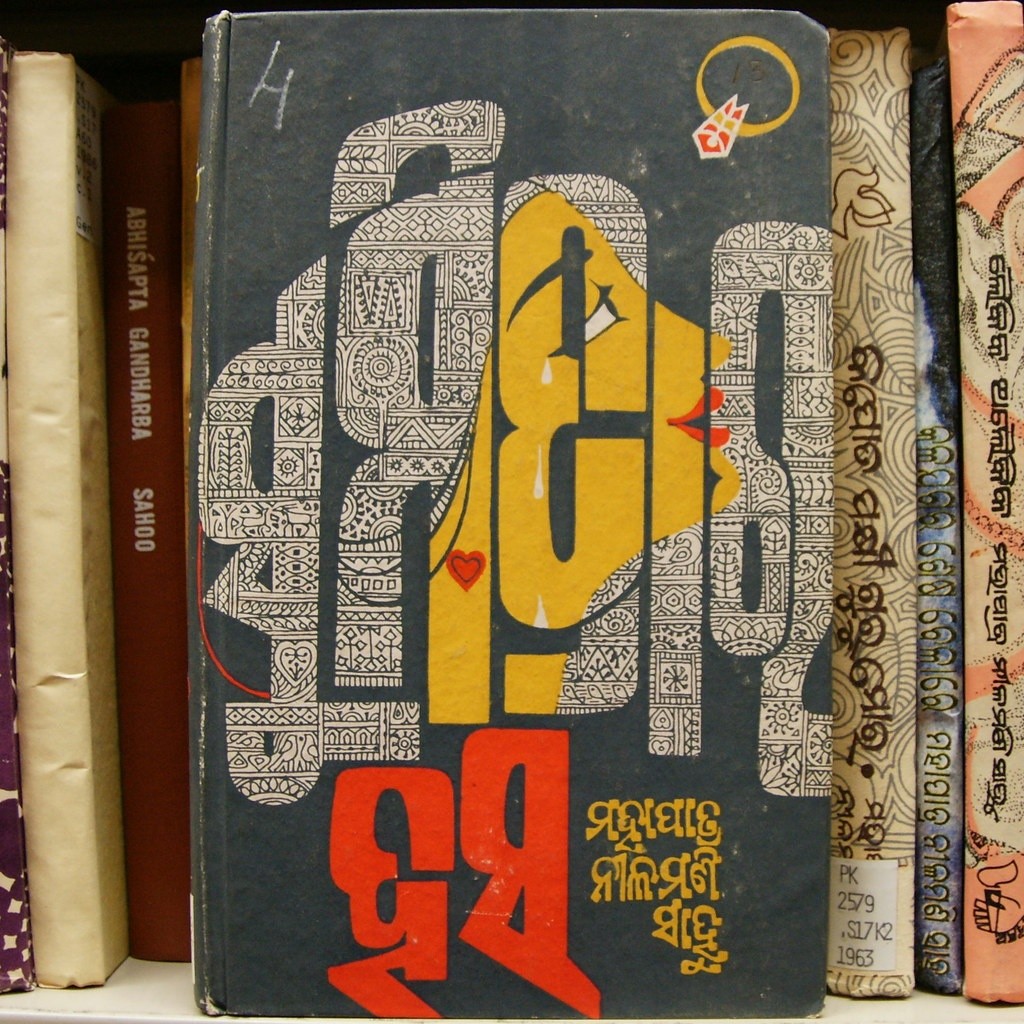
[1,9,836,1014]
[944,1,1023,1004]
[913,3,966,991]
[820,23,917,999]
